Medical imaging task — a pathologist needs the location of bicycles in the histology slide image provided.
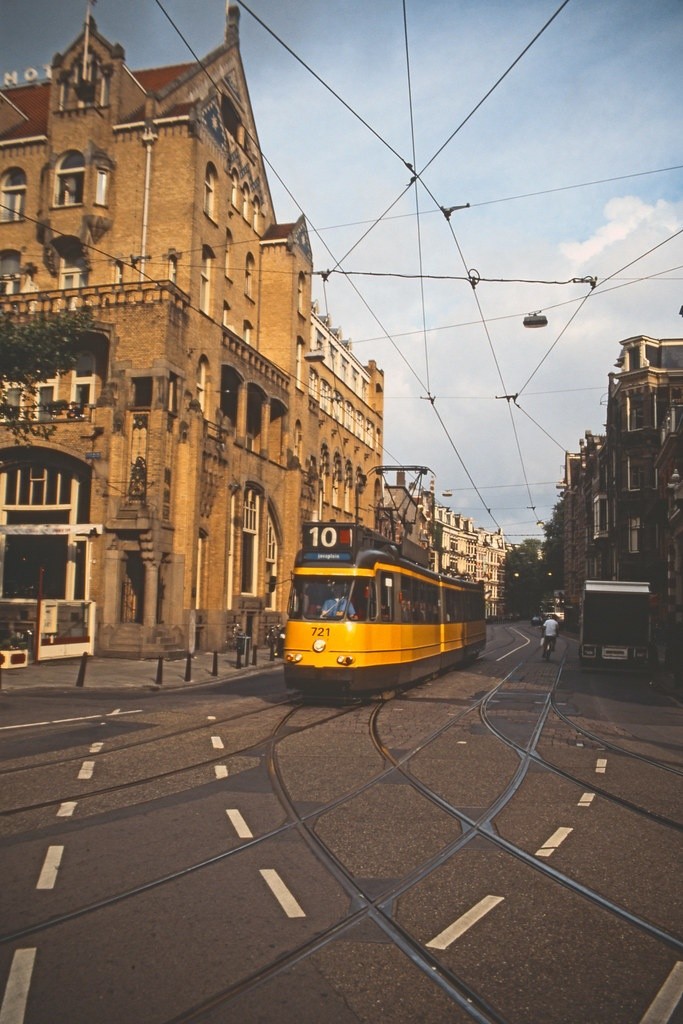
[542,629,560,661]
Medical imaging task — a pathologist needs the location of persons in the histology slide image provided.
[540,614,560,657]
[303,580,326,614]
[320,582,356,619]
[400,600,439,624]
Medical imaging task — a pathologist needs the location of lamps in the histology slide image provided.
[305,352,325,361]
[522,313,548,327]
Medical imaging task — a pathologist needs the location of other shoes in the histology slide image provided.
[542,654,546,658]
[551,647,555,652]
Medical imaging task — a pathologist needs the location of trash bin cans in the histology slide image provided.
[235,637,249,654]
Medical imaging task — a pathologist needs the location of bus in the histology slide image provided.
[578,578,653,674]
[265,465,487,706]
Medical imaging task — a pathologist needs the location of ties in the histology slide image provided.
[336,600,341,611]
